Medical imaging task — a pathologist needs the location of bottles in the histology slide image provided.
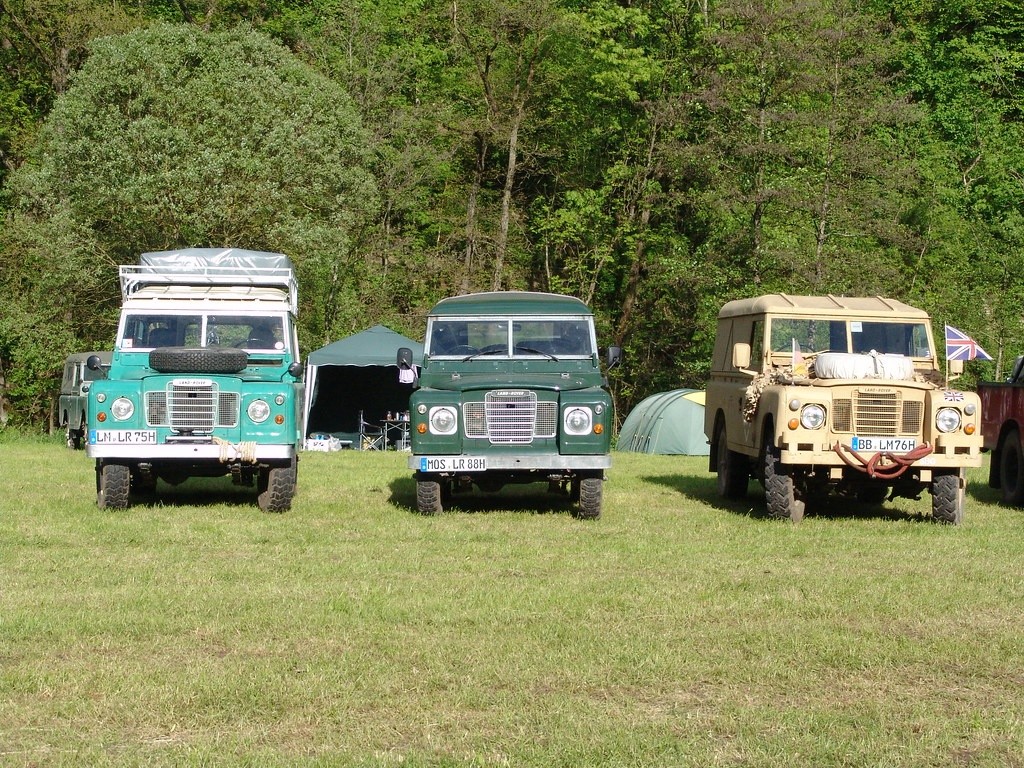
[387,411,391,420]
[396,412,399,420]
[400,410,410,420]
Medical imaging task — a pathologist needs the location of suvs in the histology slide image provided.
[58,350,115,450]
[703,294,987,527]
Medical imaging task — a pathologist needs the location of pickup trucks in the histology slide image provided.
[85,244,307,516]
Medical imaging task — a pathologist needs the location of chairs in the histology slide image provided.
[358,409,385,452]
[148,328,172,347]
[247,326,278,349]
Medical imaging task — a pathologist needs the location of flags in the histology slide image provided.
[946,326,994,360]
[796,341,802,364]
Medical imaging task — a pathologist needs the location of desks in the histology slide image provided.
[379,419,410,451]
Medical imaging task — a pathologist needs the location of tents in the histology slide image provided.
[300,325,423,449]
[616,389,709,456]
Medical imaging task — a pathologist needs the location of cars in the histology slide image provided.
[395,289,622,522]
